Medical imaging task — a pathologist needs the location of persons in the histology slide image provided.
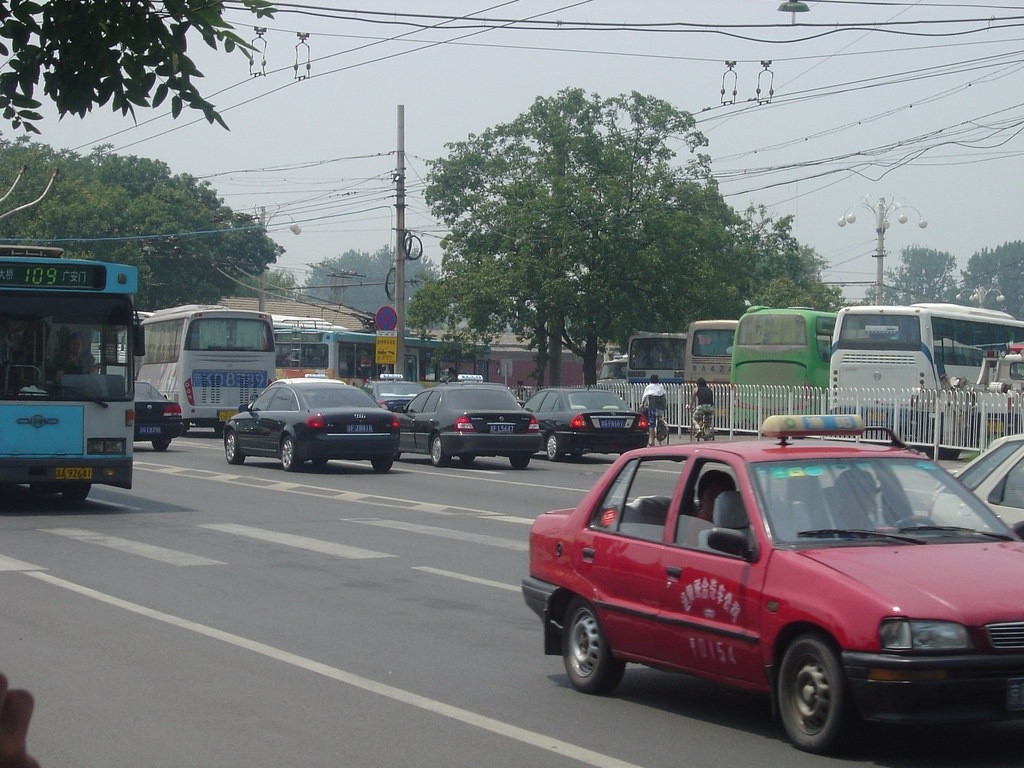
[0,319,42,387]
[686,469,735,546]
[639,373,667,446]
[830,467,882,530]
[445,370,456,381]
[684,376,716,440]
[53,330,100,383]
[514,380,529,408]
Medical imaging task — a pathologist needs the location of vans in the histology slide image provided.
[595,354,629,396]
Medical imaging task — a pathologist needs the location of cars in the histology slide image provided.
[521,413,1024,753]
[358,373,426,413]
[221,382,402,474]
[248,378,349,411]
[392,386,544,469]
[431,374,527,408]
[519,388,650,462]
[121,381,186,452]
[927,431,1024,536]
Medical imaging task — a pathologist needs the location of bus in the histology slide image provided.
[134,304,277,440]
[273,329,502,391]
[92,310,157,373]
[626,330,688,408]
[730,305,837,427]
[683,319,740,420]
[0,245,145,499]
[829,303,1024,442]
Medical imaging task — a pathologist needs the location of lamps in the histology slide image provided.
[777,0,811,13]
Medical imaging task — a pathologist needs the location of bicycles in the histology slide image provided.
[688,407,718,444]
[636,404,670,447]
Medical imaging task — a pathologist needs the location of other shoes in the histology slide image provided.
[694,429,702,438]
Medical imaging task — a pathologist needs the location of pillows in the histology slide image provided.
[602,406,620,410]
[571,404,587,410]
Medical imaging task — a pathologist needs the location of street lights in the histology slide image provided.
[837,191,928,305]
[955,285,1005,309]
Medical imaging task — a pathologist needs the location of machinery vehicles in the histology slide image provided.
[935,341,1024,458]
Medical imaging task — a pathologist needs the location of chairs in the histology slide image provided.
[699,489,757,559]
[725,346,732,354]
[1011,373,1023,379]
[787,476,822,509]
[819,485,846,538]
